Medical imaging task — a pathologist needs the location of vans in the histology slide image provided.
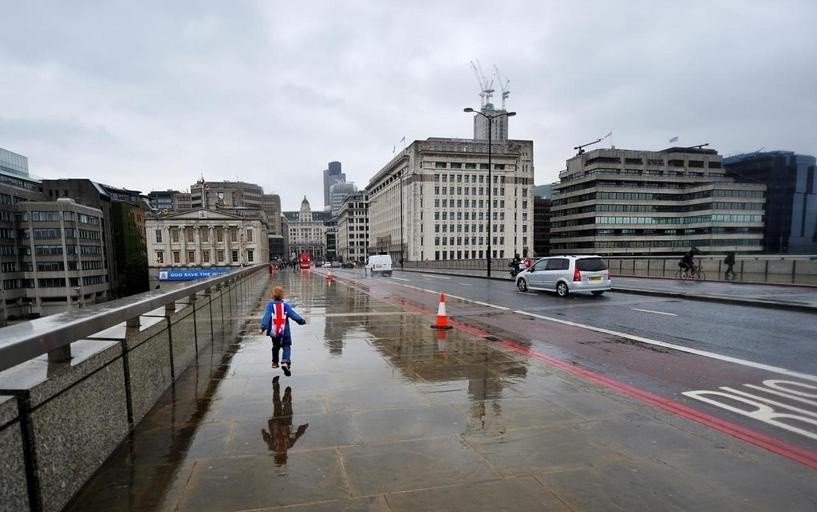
[364,255,392,276]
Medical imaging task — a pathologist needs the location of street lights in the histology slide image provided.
[463,107,516,277]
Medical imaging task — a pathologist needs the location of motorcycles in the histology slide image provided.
[508,260,524,277]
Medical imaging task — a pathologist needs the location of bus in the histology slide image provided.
[299,255,310,268]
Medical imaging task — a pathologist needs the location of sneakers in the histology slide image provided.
[272,362,291,376]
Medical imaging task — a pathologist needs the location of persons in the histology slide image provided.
[274,255,298,270]
[725,253,735,277]
[260,287,305,376]
[678,251,695,277]
[261,376,309,467]
[512,254,521,274]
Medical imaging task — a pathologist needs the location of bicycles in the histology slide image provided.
[675,264,706,283]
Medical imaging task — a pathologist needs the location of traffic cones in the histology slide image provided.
[434,293,448,327]
[326,271,332,279]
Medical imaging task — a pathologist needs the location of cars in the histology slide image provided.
[314,260,353,268]
[514,254,611,297]
